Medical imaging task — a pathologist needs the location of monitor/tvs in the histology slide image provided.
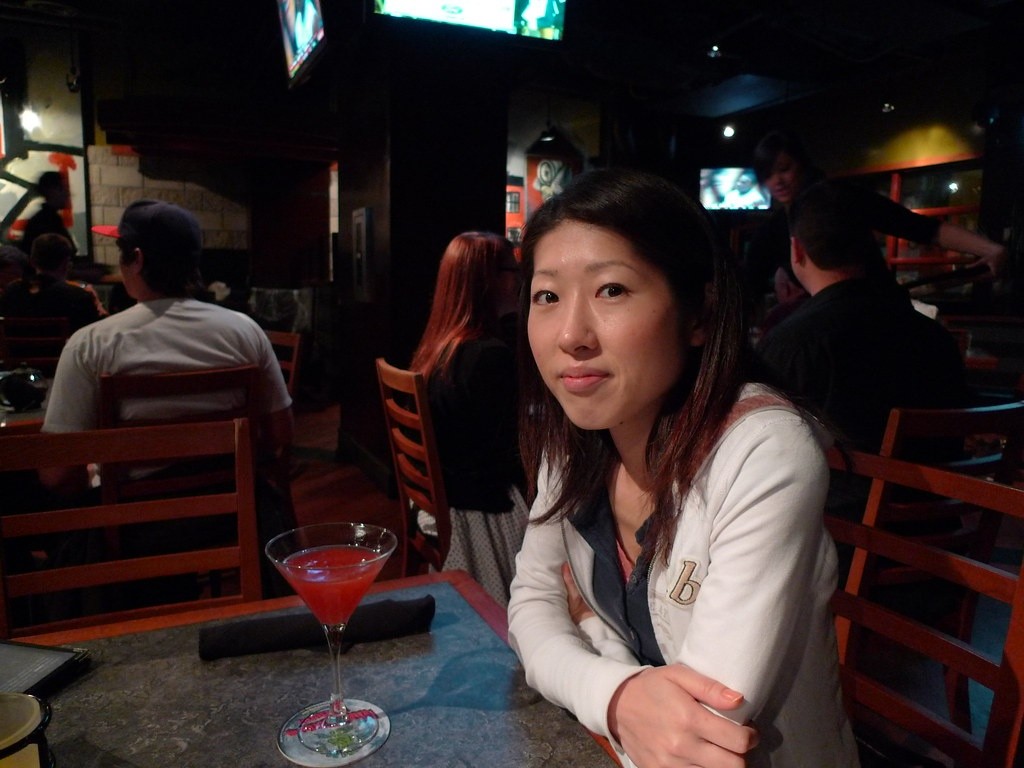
[278,0,330,90]
[363,0,573,55]
[698,166,772,211]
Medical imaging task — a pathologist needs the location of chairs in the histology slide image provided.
[831,400,1024,733]
[0,316,69,370]
[263,329,303,396]
[823,447,1024,768]
[0,417,262,641]
[97,363,262,557]
[375,356,451,577]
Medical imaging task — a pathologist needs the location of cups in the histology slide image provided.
[0,692,43,768]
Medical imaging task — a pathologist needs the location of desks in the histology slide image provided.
[0,377,54,425]
[11,569,622,768]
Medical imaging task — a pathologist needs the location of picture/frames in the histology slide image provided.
[351,207,371,291]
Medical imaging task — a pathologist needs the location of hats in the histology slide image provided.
[91,199,201,251]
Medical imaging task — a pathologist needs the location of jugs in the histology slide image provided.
[0,361,48,412]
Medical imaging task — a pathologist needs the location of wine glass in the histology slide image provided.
[263,522,398,756]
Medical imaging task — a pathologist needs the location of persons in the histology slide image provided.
[0,172,232,377]
[503,168,860,768]
[34,200,296,554]
[400,121,1024,627]
